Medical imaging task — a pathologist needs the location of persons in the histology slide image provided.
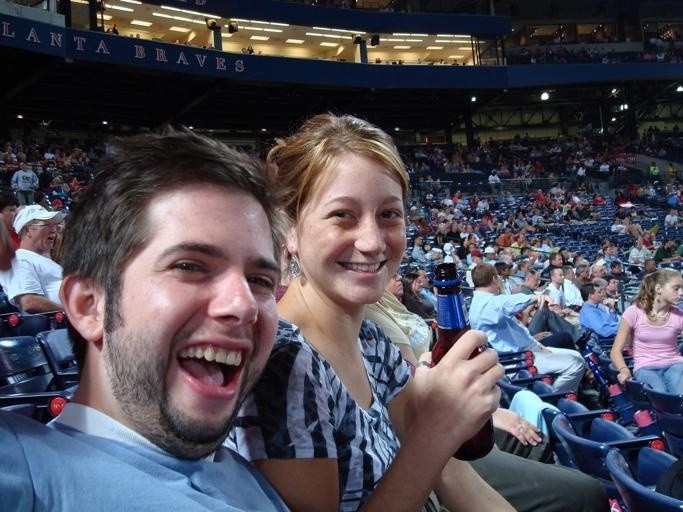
[0,130,289,512]
[175,40,255,54]
[375,58,459,64]
[527,38,681,65]
[107,24,119,35]
[218,113,516,512]
[363,122,683,512]
[1,134,104,316]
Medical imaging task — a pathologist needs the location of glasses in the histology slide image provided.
[29,223,57,230]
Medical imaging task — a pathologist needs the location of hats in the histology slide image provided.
[14,204,62,234]
[484,246,495,254]
[470,249,480,257]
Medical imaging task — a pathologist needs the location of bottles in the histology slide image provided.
[430,263,496,462]
